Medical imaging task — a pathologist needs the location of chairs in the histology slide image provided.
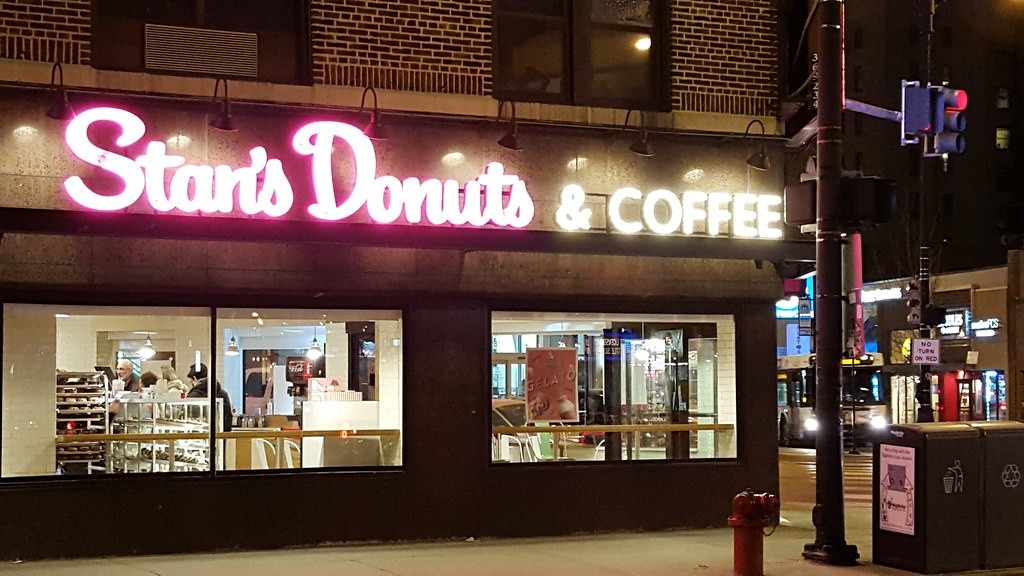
[492,422,604,462]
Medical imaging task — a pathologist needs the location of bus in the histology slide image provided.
[777,351,888,445]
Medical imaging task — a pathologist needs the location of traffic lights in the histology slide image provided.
[903,84,946,138]
[850,176,897,225]
[929,307,946,324]
[936,88,967,155]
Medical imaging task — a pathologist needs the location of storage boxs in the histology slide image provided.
[323,392,363,401]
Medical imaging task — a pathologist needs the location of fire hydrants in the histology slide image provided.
[725,488,778,576]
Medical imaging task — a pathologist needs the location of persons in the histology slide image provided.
[841,376,853,396]
[187,363,233,433]
[140,364,189,410]
[108,359,143,413]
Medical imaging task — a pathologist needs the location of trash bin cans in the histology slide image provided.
[873,421,982,575]
[962,421,1024,571]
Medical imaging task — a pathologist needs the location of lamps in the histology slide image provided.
[210,77,238,133]
[226,320,240,355]
[558,322,567,347]
[743,119,771,171]
[353,86,387,140]
[613,108,655,157]
[307,327,320,359]
[495,98,519,149]
[139,320,154,359]
[46,63,77,120]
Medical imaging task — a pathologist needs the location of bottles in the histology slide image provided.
[242,414,249,428]
[248,408,265,428]
[237,414,242,427]
[231,408,237,426]
[267,400,273,415]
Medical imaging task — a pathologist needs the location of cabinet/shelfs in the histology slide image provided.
[108,392,225,472]
[56,371,108,474]
